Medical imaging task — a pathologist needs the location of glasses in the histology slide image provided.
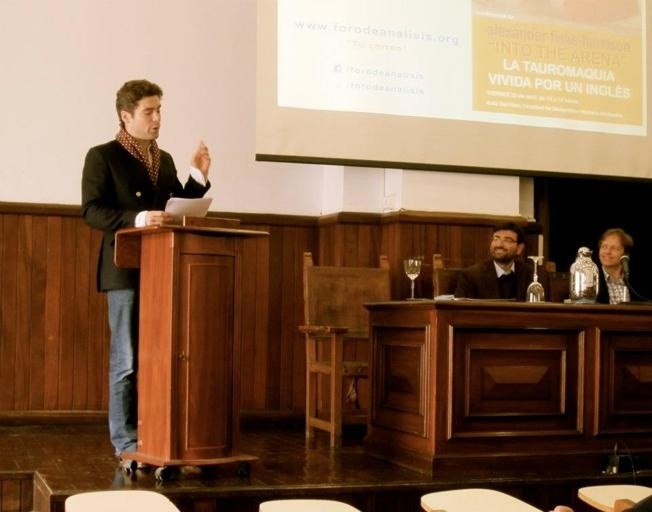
[493,234,517,243]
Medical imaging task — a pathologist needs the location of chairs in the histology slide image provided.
[295,246,572,450]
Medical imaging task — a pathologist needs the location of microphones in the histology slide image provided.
[619,254,630,277]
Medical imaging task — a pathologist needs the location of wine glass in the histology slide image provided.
[403,259,422,300]
[524,256,546,302]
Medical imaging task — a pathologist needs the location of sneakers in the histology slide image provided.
[117,457,146,470]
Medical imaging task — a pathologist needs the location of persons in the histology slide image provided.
[83,80,212,471]
[596,228,652,304]
[454,222,552,302]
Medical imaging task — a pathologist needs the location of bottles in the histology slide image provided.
[569,247,600,304]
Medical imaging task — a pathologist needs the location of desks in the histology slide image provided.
[360,295,650,475]
